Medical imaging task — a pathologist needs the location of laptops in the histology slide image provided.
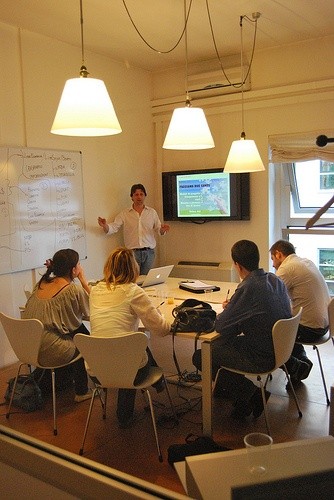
[136,265,174,287]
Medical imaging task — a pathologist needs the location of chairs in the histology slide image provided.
[0,298,334,464]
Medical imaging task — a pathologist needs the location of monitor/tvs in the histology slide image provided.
[172,172,238,217]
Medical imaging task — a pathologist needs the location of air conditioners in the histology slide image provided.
[185,63,253,100]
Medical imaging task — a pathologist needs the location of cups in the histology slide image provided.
[204,289,213,300]
[152,290,174,304]
[243,433,273,476]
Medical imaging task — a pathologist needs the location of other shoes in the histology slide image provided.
[231,406,251,417]
[118,419,131,427]
[285,360,312,390]
[74,388,100,402]
[252,388,271,417]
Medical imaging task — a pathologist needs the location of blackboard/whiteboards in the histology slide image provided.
[0,145,88,275]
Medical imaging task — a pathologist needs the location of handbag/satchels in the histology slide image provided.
[4,373,42,410]
[169,298,216,332]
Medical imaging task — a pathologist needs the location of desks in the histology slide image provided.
[185,435,334,500]
[19,275,241,441]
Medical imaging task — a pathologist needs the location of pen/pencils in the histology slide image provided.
[226,289,230,302]
[156,301,165,309]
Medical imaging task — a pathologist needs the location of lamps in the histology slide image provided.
[49,0,123,138]
[162,0,216,150]
[223,16,266,174]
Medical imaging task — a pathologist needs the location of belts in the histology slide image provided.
[135,248,149,251]
[299,324,328,336]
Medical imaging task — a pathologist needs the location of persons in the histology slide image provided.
[269,240,331,390]
[98,184,169,276]
[88,247,171,429]
[192,240,293,417]
[23,249,91,403]
